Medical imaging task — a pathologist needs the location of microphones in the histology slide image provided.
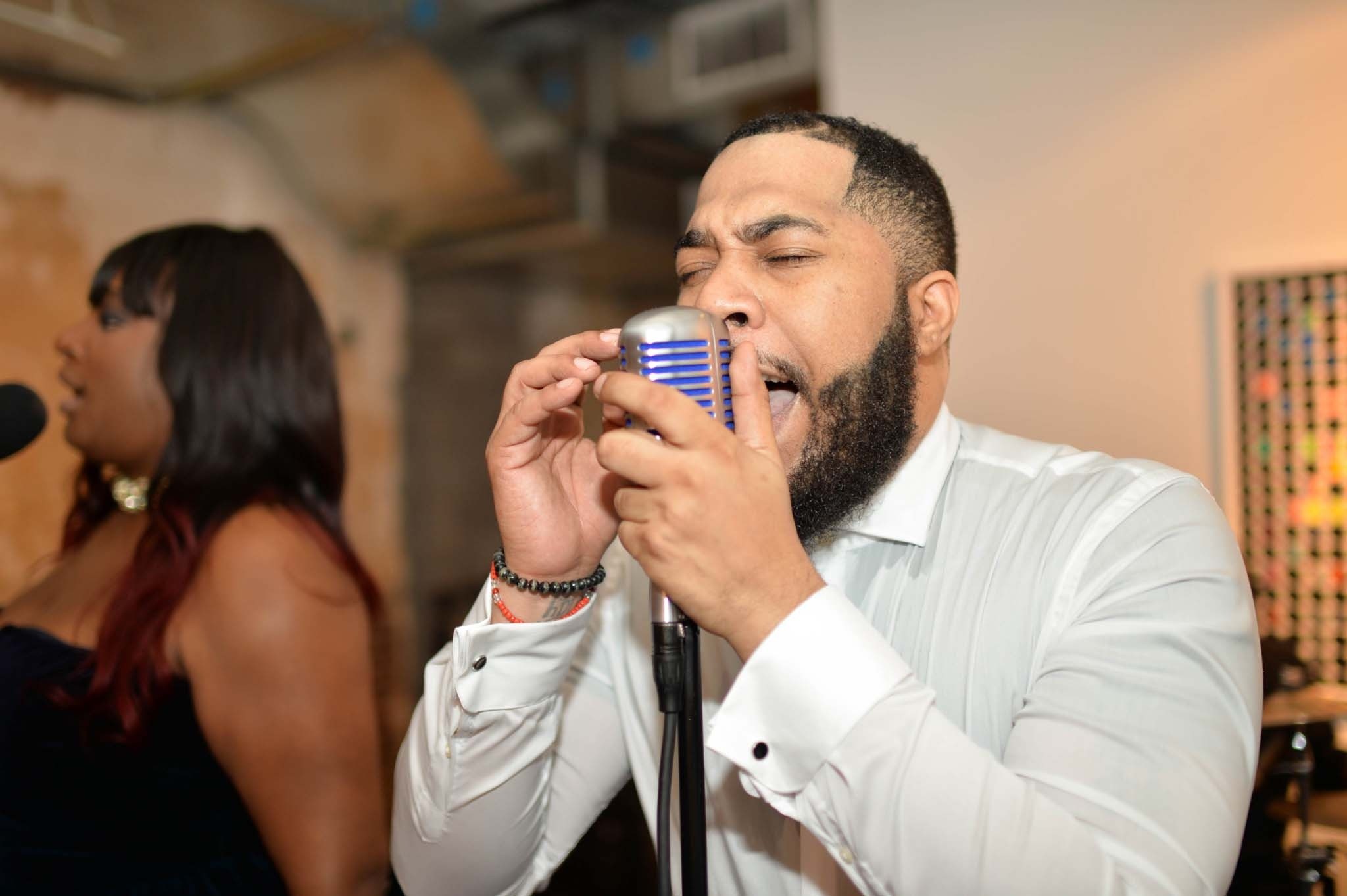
[619,307,736,445]
[0,382,49,460]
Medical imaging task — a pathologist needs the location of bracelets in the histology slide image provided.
[494,543,606,595]
[489,557,597,625]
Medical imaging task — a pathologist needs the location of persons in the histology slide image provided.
[0,221,402,896]
[389,104,1265,896]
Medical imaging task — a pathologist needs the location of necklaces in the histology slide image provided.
[104,468,152,513]
